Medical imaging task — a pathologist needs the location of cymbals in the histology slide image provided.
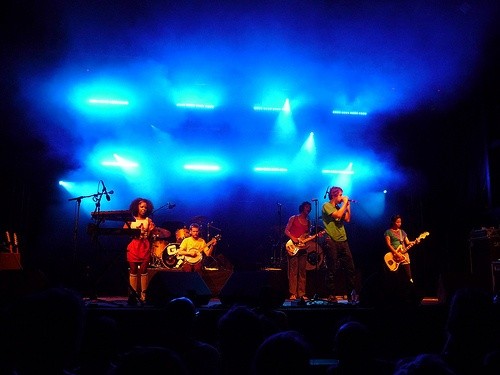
[152,227,170,238]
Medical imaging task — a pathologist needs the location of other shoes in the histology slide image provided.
[289,295,308,300]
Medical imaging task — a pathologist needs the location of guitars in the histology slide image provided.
[384,231,430,272]
[186,233,221,265]
[286,228,327,256]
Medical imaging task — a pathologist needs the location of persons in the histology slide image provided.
[383,215,421,285]
[122,197,157,302]
[322,187,358,303]
[284,201,324,301]
[177,223,217,279]
[0,289,500,375]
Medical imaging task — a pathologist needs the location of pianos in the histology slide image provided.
[87,211,141,305]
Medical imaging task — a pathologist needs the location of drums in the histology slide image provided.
[161,243,185,270]
[150,240,168,257]
[305,242,323,270]
[175,229,190,242]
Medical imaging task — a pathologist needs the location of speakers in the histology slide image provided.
[145,271,211,302]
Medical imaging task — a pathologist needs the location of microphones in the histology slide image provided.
[341,199,356,202]
[311,199,318,201]
[101,182,110,201]
[168,204,176,208]
